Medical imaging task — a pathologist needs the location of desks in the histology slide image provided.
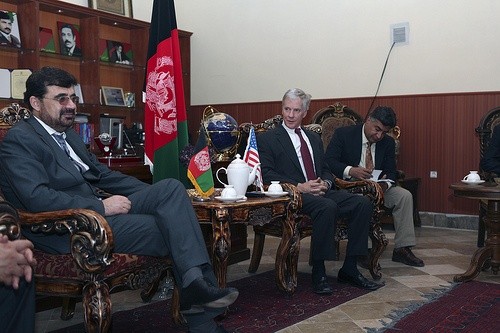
[188,188,298,293]
[450,180,500,282]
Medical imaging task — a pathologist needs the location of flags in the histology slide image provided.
[187,128,214,198]
[144,0,187,185]
[244,128,262,182]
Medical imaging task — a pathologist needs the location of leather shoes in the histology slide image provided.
[178,276,238,315]
[187,317,228,333]
[357,248,381,270]
[392,247,424,267]
[311,267,333,293]
[336,269,381,290]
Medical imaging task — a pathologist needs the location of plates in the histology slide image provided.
[214,197,244,202]
[265,192,289,197]
[461,180,485,183]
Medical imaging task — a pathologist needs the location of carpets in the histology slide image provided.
[367,278,500,333]
[49,270,385,333]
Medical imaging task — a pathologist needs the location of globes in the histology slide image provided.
[198,105,243,162]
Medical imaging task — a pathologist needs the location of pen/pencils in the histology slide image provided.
[358,164,373,178]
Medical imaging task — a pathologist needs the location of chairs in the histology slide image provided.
[240,115,388,290]
[310,104,422,228]
[475,108,500,246]
[0,102,189,333]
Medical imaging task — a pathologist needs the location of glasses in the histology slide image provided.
[35,95,80,106]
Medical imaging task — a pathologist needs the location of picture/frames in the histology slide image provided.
[102,86,126,106]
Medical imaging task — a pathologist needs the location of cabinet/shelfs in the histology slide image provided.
[0,0,190,158]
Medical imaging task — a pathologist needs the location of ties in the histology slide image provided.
[52,133,86,174]
[295,127,317,181]
[365,142,374,171]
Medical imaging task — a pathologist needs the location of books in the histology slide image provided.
[362,169,395,183]
[72,113,95,150]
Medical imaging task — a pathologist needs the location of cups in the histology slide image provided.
[463,171,481,181]
[268,181,283,193]
[220,185,236,197]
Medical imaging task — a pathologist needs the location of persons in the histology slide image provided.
[61,25,81,56]
[111,44,130,64]
[112,91,123,104]
[0,67,238,333]
[256,90,380,294]
[481,125,500,181]
[0,234,37,333]
[327,107,424,270]
[0,11,19,45]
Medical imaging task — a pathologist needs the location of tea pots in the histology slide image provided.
[216,154,262,203]
